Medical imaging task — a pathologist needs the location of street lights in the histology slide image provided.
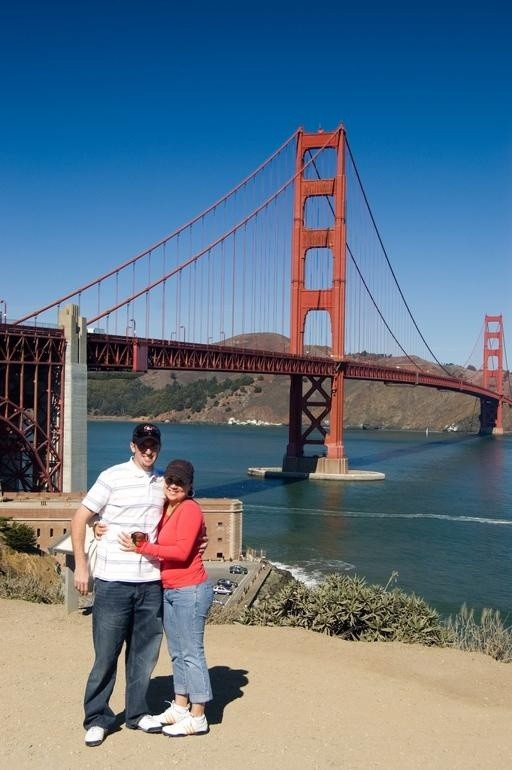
[207,336,213,344]
[180,325,186,345]
[130,319,136,337]
[1,299,8,324]
[126,326,133,335]
[171,332,176,339]
[220,331,226,345]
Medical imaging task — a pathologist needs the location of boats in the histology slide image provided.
[442,424,457,432]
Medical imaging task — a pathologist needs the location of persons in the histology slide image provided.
[70,424,209,747]
[94,458,214,740]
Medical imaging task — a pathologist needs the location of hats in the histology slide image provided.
[164,459,195,484]
[133,423,161,446]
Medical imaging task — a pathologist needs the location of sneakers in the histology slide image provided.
[138,713,162,733]
[84,725,108,747]
[152,700,190,725]
[162,709,209,735]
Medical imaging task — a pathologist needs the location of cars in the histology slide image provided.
[212,585,233,596]
[229,565,248,574]
[217,577,238,587]
[213,597,224,605]
